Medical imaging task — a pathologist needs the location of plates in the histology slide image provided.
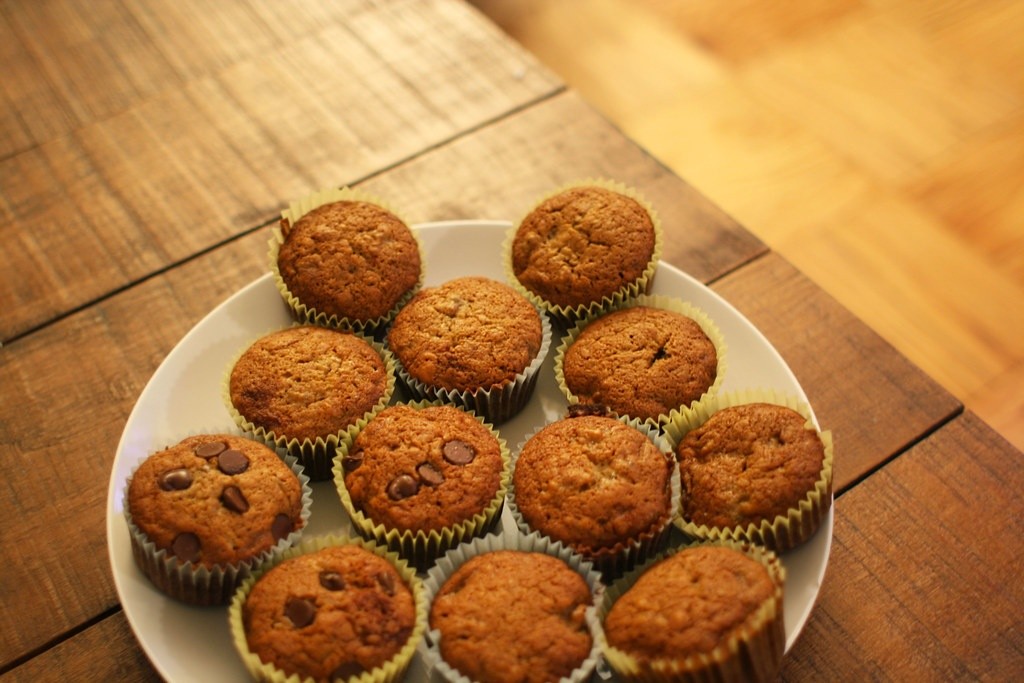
[101,219,832,683]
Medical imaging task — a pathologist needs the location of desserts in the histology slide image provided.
[119,175,832,683]
